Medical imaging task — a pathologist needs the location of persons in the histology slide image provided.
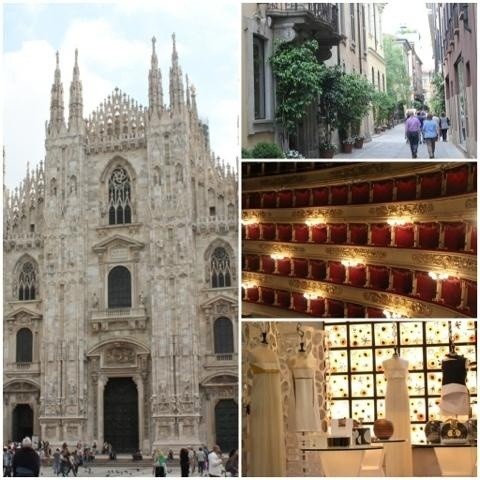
[381,347,414,477]
[131,449,143,460]
[439,112,451,143]
[245,332,288,476]
[284,341,322,434]
[405,111,421,157]
[421,114,438,159]
[404,110,440,145]
[206,445,226,477]
[179,447,192,477]
[439,343,472,417]
[226,448,238,477]
[182,443,210,475]
[152,455,168,477]
[3,436,117,477]
[152,447,175,464]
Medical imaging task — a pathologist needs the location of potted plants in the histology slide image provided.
[317,65,373,158]
